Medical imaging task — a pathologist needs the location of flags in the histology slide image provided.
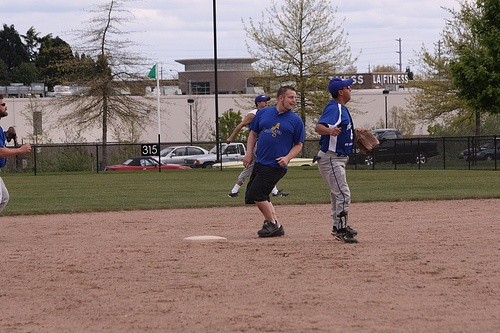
[148,64,156,79]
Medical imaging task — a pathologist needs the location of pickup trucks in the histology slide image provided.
[349,128,441,166]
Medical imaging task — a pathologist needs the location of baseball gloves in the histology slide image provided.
[4,126,17,142]
[355,128,380,151]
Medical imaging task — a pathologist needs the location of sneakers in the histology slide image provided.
[228,192,239,198]
[331,226,358,235]
[273,189,289,197]
[335,231,358,244]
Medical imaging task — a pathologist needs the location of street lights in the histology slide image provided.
[382,90,390,130]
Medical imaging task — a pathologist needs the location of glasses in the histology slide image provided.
[340,85,350,90]
[0,103,6,106]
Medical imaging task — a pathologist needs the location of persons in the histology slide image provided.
[0,95,32,216]
[315,77,380,243]
[243,86,305,237]
[226,94,290,197]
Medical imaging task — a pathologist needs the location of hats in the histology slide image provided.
[255,94,270,102]
[328,77,353,95]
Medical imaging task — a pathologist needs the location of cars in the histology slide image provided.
[153,146,217,168]
[104,157,192,171]
[458,138,500,160]
[212,157,318,168]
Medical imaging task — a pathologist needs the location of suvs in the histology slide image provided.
[183,142,247,168]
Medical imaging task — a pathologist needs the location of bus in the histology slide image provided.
[0,82,47,99]
[46,85,94,98]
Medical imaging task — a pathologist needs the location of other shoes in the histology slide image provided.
[257,220,284,238]
